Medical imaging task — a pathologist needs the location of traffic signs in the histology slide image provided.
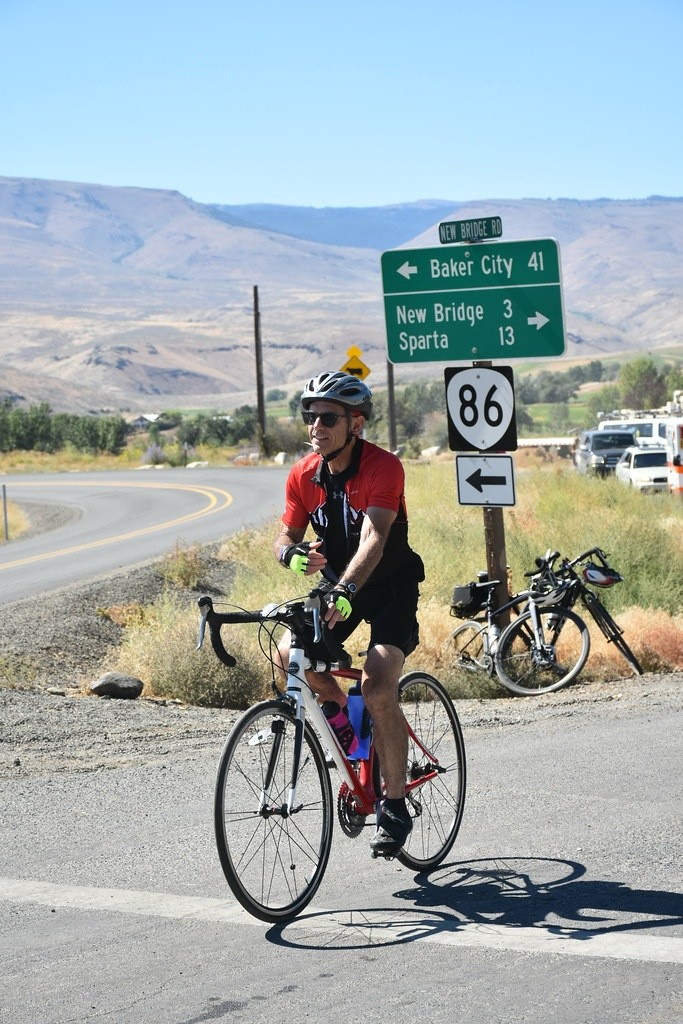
[380,235,567,368]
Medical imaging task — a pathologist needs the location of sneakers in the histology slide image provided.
[369,805,415,851]
[319,720,355,768]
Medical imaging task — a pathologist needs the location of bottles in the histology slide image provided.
[346,681,370,760]
[488,624,501,656]
[545,602,561,630]
[321,700,359,755]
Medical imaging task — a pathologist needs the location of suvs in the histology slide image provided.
[574,426,642,477]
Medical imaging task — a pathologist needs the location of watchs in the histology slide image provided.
[338,580,357,599]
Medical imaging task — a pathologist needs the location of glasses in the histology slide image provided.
[301,411,361,427]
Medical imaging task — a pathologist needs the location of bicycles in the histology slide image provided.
[438,549,593,696]
[474,542,643,676]
[194,587,466,924]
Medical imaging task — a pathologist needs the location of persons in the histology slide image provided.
[274,371,422,850]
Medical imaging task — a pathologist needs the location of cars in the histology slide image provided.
[614,442,670,494]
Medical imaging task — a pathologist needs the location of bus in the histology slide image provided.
[595,392,683,445]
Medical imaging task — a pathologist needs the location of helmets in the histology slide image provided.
[300,370,373,406]
[581,559,625,588]
